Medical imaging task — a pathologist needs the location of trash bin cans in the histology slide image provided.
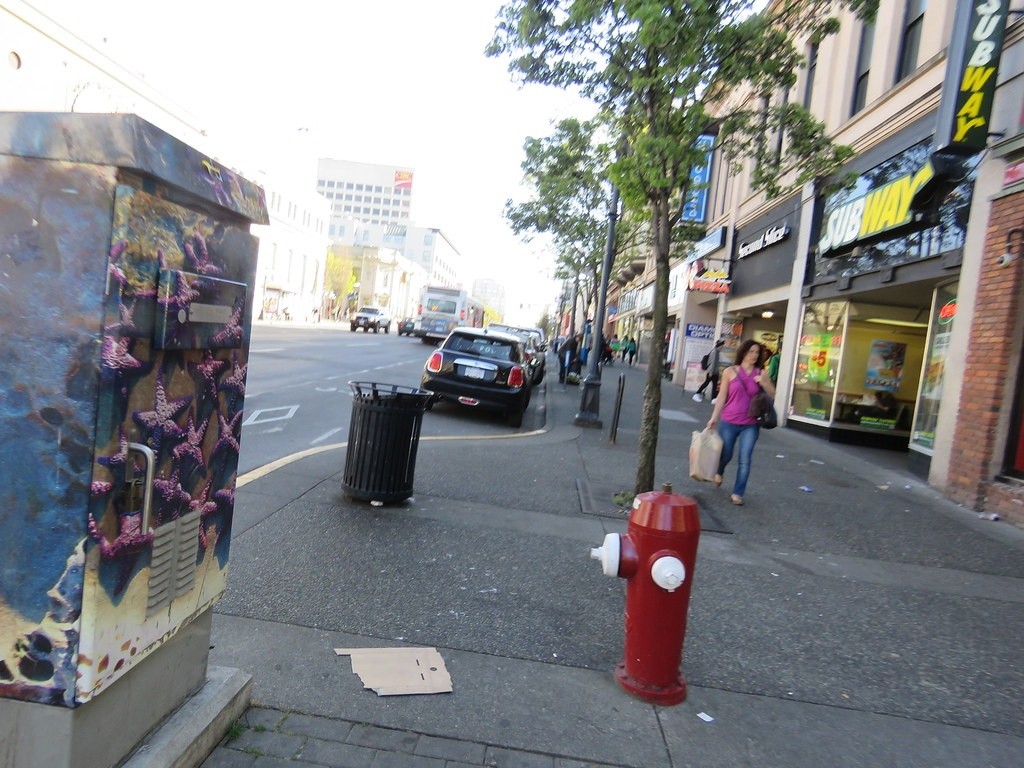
[339,379,435,505]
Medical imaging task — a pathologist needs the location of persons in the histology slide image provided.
[692,339,725,405]
[599,333,619,366]
[758,340,783,422]
[706,341,776,505]
[557,334,583,384]
[626,338,637,366]
[621,335,629,364]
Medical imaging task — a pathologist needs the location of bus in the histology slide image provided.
[414,285,485,346]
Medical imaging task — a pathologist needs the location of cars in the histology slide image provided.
[420,326,541,429]
[398,317,418,337]
[487,322,547,385]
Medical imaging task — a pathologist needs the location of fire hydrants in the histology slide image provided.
[588,479,701,707]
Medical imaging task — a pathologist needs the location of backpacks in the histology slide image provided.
[701,349,715,370]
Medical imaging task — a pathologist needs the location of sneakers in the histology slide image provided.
[711,399,717,405]
[691,394,702,402]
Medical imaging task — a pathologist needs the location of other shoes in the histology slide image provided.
[715,482,721,487]
[729,495,746,506]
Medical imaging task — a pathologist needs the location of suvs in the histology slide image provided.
[350,305,392,334]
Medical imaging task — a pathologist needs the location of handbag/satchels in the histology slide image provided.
[689,428,723,483]
[757,405,778,429]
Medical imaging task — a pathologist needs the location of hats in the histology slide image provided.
[750,395,769,417]
